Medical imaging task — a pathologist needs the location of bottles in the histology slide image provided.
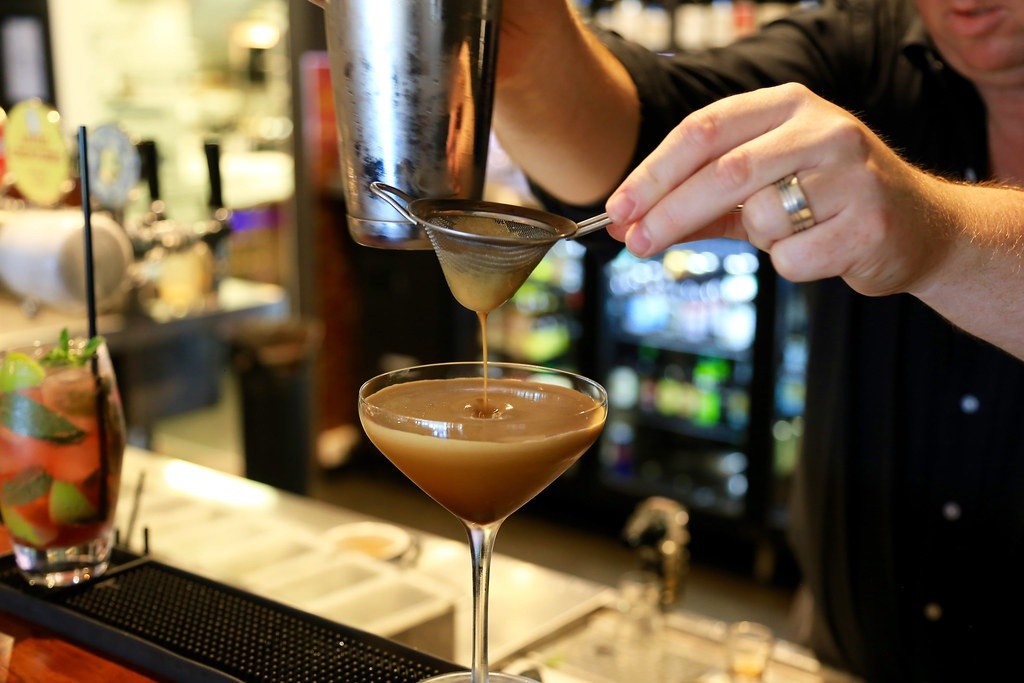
[699,621,774,683]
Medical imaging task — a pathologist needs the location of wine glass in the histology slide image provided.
[359,360,609,683]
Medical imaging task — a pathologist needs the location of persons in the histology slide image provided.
[309,0,1024,683]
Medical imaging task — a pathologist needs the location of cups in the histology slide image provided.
[0,338,127,589]
[324,0,503,252]
[326,520,410,562]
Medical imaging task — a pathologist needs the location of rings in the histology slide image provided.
[778,173,817,233]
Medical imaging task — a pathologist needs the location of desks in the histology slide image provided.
[107,277,289,414]
[113,444,616,669]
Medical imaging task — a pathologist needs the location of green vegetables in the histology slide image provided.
[0,328,106,546]
